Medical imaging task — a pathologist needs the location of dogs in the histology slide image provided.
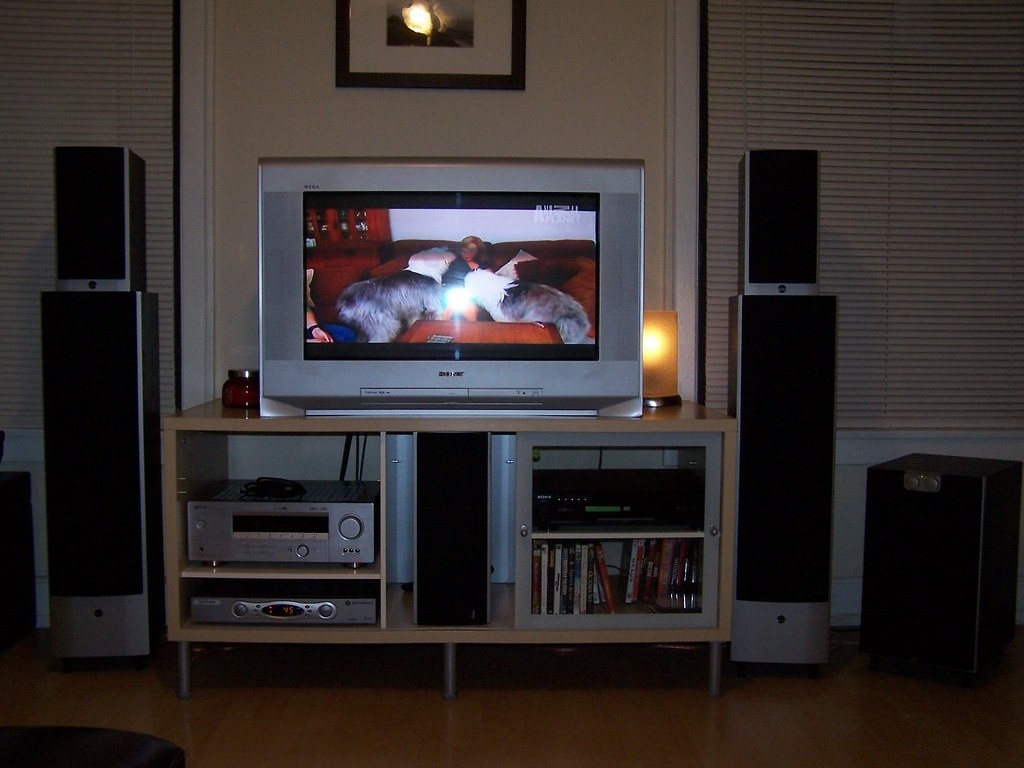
[335,247,452,343]
[464,269,591,344]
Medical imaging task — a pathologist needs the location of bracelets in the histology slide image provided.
[308,325,319,332]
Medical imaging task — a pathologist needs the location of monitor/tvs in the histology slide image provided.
[256,157,644,418]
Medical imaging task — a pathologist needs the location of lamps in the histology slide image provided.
[642,310,680,406]
[400,0,434,35]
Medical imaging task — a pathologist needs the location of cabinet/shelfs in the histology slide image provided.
[157,401,739,701]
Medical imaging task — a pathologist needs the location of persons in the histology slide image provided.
[306,268,358,343]
[441,237,492,321]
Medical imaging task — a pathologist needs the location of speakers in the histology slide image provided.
[874,451,1022,685]
[41,145,165,666]
[0,471,38,654]
[411,431,490,628]
[730,149,840,668]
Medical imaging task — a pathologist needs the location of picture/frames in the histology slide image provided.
[335,0,525,92]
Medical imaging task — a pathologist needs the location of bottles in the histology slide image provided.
[222,368,259,409]
